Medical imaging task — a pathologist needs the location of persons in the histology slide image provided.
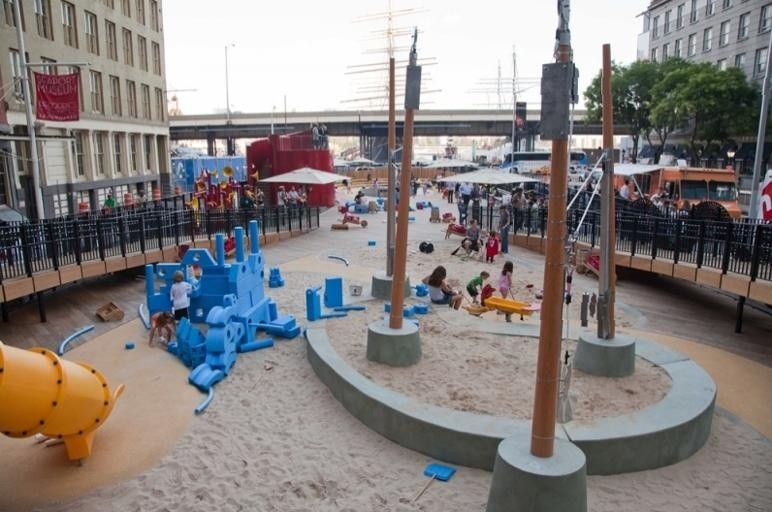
[620,177,630,199]
[276,184,308,210]
[420,264,464,310]
[630,185,640,201]
[334,159,550,263]
[103,193,118,223]
[135,189,148,203]
[466,271,490,304]
[147,310,173,348]
[496,261,514,315]
[650,186,689,237]
[255,187,265,208]
[169,271,194,325]
[244,185,255,208]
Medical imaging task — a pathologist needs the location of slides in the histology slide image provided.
[0,342,126,461]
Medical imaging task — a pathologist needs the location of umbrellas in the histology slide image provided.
[258,164,349,209]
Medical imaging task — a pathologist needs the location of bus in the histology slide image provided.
[496,149,589,177]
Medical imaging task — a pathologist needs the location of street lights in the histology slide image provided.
[224,41,238,156]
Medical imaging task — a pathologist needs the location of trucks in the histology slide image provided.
[614,163,742,222]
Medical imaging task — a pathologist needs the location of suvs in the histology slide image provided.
[0,203,31,256]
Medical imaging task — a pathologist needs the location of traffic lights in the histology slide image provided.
[515,101,528,133]
